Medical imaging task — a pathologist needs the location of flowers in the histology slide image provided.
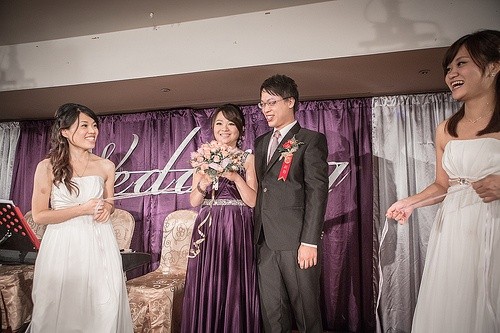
[277,134,305,161]
[190,140,247,191]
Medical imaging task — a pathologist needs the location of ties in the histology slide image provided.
[268,130,281,164]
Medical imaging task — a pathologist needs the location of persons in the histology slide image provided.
[253,74,329,333]
[30,103,134,333]
[181,103,261,333]
[386,29,500,333]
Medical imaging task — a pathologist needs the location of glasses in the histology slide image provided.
[258,96,293,108]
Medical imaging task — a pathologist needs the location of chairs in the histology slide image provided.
[0,211,48,333]
[111,208,135,251]
[126,209,198,333]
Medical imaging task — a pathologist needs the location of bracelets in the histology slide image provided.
[197,182,206,194]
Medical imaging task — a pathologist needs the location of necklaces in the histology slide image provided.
[465,109,492,124]
[70,156,90,178]
